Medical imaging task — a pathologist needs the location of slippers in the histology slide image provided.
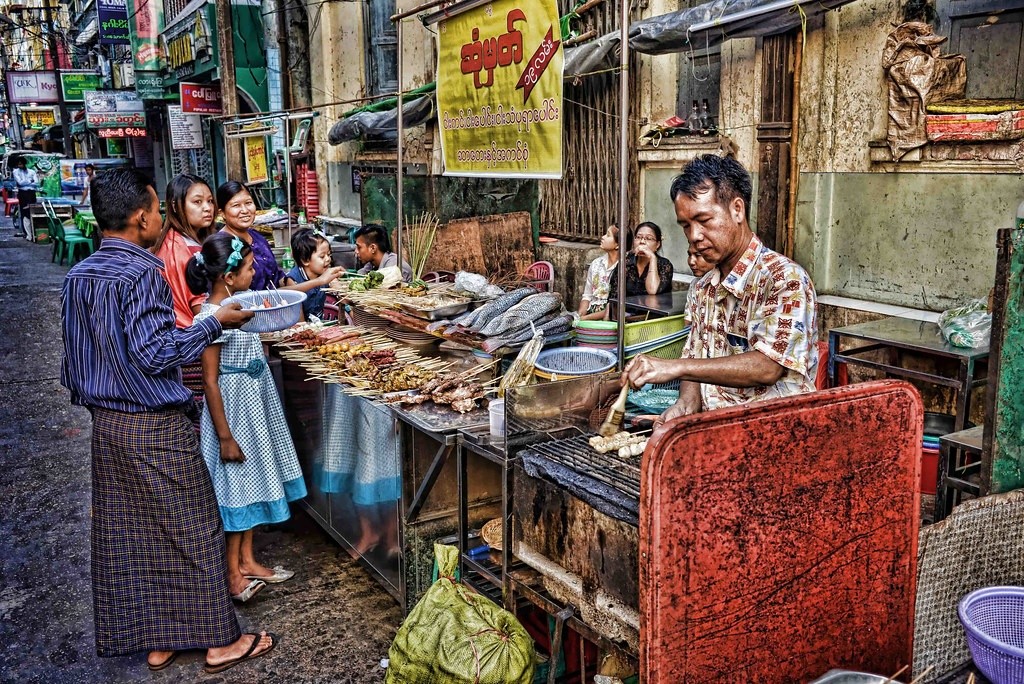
[147,650,184,670]
[203,632,277,674]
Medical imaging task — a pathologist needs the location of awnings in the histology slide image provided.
[24,131,45,142]
[68,119,100,144]
[42,123,73,141]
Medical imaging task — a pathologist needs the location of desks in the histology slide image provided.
[826,316,989,507]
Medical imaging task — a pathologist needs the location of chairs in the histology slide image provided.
[1,188,19,217]
[42,199,93,265]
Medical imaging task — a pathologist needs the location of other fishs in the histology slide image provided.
[425,287,575,355]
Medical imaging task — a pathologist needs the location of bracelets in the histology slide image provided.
[648,270,658,273]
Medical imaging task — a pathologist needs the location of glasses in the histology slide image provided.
[633,235,657,242]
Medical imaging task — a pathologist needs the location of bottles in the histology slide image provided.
[282,247,295,275]
[688,99,702,134]
[699,99,717,135]
[298,212,306,225]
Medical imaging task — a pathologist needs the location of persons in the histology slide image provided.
[352,223,413,283]
[13,157,38,238]
[620,153,819,432]
[60,166,281,673]
[578,221,673,321]
[79,163,97,206]
[146,174,331,445]
[684,240,715,326]
[185,232,347,604]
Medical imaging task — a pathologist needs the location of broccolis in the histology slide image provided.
[349,271,384,291]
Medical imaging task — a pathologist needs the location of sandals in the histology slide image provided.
[230,579,266,603]
[347,541,378,560]
[244,566,295,583]
[387,546,403,559]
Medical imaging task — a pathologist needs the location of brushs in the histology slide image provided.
[599,380,631,436]
